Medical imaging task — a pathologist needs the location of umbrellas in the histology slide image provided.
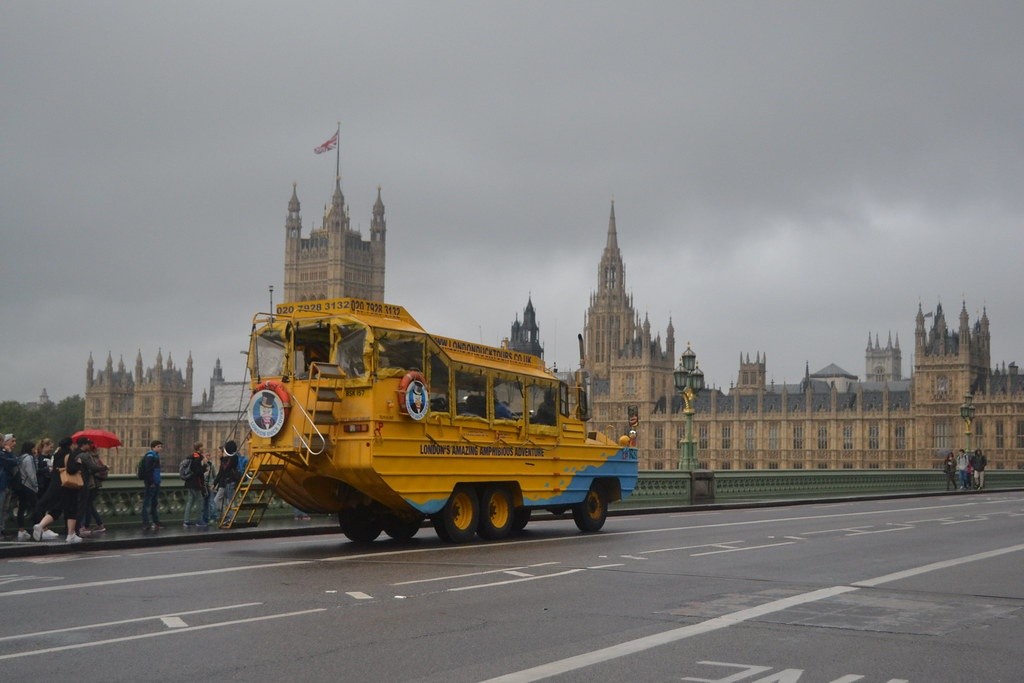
[72,429,123,449]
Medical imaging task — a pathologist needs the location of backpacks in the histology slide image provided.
[178,456,196,480]
[137,451,156,479]
[8,455,29,490]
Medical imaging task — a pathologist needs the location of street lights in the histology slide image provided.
[960,392,976,455]
[673,338,703,467]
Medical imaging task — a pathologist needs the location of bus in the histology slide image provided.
[217,296,640,546]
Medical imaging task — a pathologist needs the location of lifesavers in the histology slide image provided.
[398,371,430,413]
[249,381,290,427]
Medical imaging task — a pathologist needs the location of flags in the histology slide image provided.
[314,132,338,154]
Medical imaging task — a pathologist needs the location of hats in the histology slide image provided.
[77,437,94,447]
[4,433,15,442]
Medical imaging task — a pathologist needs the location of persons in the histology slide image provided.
[945,452,957,490]
[481,389,513,420]
[211,446,237,526]
[530,388,556,426]
[956,449,970,490]
[297,357,321,380]
[0,433,109,543]
[183,442,216,527]
[971,449,987,491]
[141,441,165,530]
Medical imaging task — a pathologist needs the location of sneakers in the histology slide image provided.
[18,531,30,539]
[66,533,82,541]
[43,529,59,538]
[34,524,41,541]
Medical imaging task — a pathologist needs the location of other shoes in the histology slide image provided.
[183,521,194,526]
[196,520,207,526]
[144,522,163,529]
[79,528,91,534]
[93,526,106,532]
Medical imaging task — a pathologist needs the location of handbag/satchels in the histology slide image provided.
[58,454,84,490]
